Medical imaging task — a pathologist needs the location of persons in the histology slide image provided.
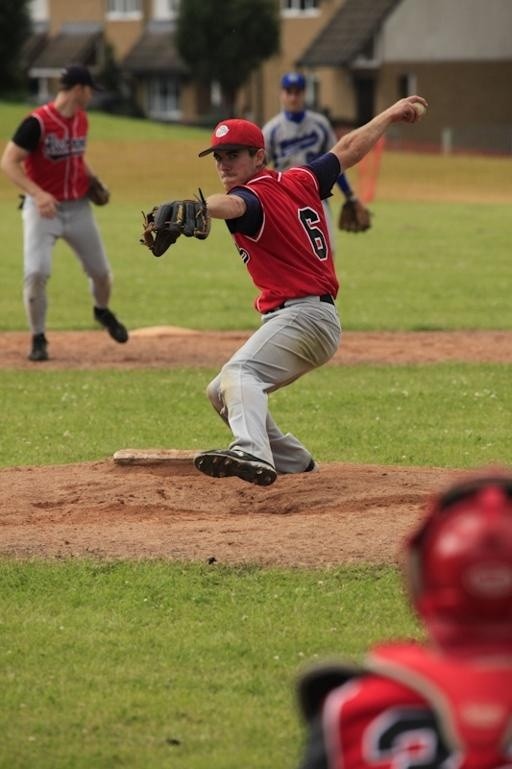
[1,59,132,364]
[138,93,428,490]
[257,70,377,279]
[289,474,511,769]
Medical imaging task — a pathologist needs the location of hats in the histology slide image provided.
[198,116,265,161]
[57,62,106,93]
[279,71,307,91]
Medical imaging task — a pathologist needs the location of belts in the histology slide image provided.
[259,292,336,316]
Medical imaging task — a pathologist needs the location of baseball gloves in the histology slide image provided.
[139,188,211,257]
[86,175,108,206]
[339,197,370,233]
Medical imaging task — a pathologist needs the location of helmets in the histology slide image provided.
[393,469,511,663]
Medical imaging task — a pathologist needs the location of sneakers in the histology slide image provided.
[92,302,130,346]
[189,446,279,489]
[25,328,52,364]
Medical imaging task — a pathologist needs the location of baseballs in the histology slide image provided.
[412,102,428,123]
